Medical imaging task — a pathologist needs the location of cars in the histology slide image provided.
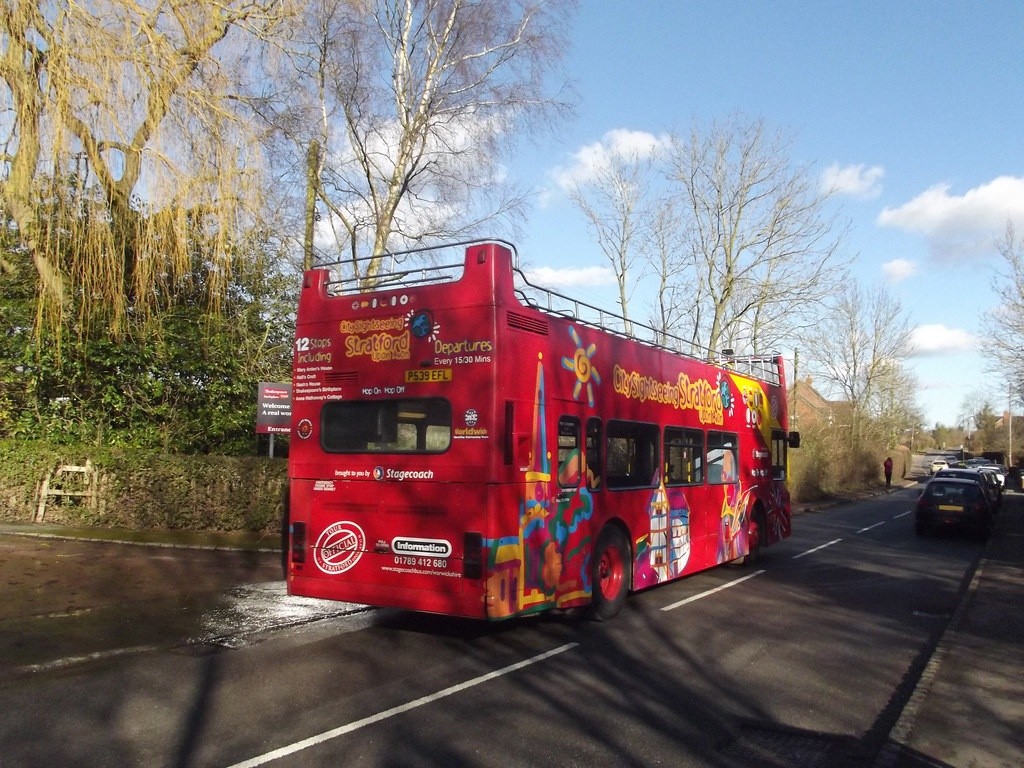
[912,457,1010,543]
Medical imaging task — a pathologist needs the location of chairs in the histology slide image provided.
[963,488,974,498]
[934,486,946,499]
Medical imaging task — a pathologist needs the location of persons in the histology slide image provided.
[884,457,893,489]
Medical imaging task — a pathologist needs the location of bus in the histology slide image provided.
[279,236,802,625]
